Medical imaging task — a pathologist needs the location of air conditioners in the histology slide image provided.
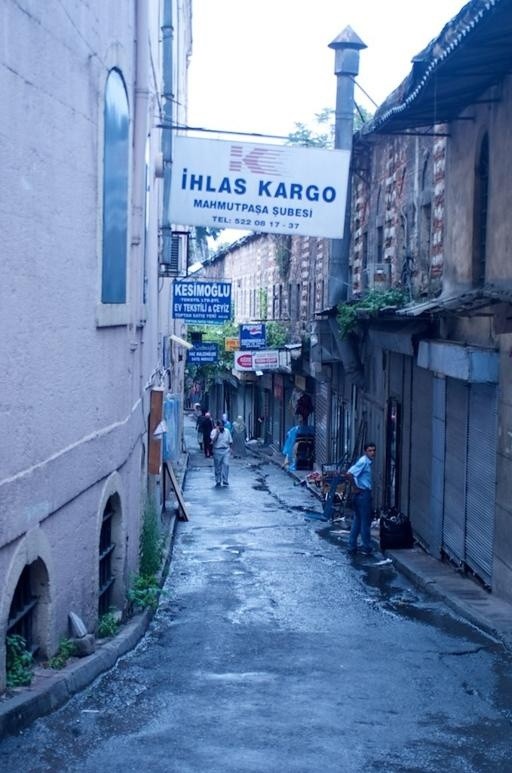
[159,235,184,275]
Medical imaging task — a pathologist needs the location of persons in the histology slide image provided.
[209,416,233,488]
[184,380,248,459]
[342,439,380,554]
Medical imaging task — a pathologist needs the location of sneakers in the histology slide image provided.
[215,481,229,487]
[348,547,377,555]
